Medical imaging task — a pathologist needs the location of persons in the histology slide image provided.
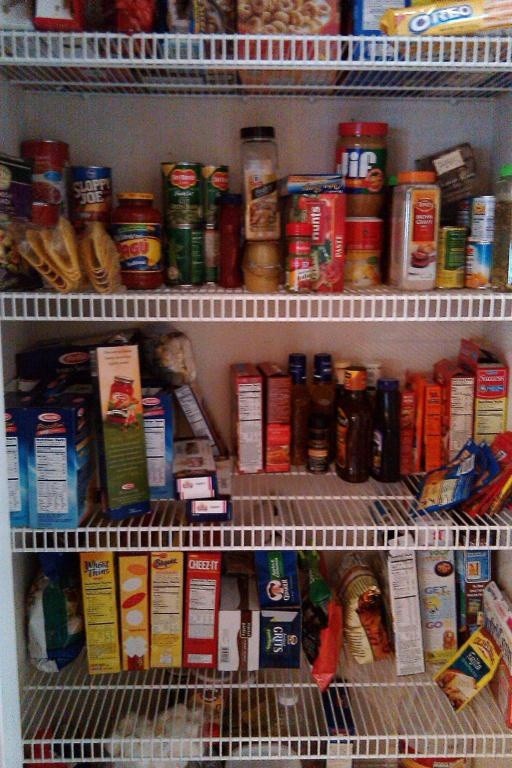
[289,635,297,645]
[267,582,283,598]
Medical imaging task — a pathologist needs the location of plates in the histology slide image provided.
[31,175,64,205]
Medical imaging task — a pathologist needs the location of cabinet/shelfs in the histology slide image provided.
[1,0,512,767]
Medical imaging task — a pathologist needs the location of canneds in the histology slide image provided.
[20,138,231,290]
[438,194,496,291]
[166,668,232,714]
[335,123,389,217]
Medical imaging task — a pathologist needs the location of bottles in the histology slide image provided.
[227,126,284,242]
[242,243,284,291]
[273,692,308,737]
[337,120,388,219]
[494,165,511,292]
[389,171,438,291]
[111,191,165,291]
[288,351,400,483]
